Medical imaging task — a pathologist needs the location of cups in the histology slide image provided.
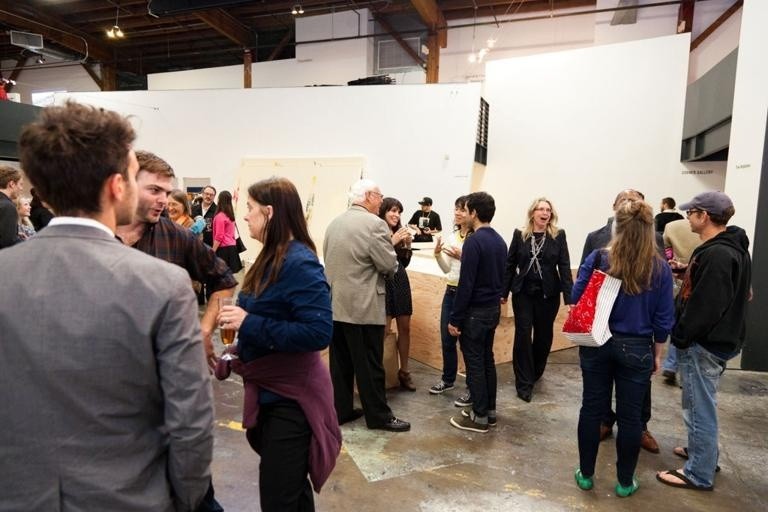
[424,223,430,232]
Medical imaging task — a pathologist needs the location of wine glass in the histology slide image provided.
[670,255,688,288]
[217,295,239,361]
[399,224,411,248]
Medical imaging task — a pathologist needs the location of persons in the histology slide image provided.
[656,191,751,491]
[576,191,660,452]
[655,198,704,386]
[571,197,677,496]
[508,198,573,403]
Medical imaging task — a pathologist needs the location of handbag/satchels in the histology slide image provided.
[562,268,623,349]
[235,235,247,253]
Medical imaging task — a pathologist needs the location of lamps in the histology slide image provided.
[290,2,304,15]
[103,6,124,39]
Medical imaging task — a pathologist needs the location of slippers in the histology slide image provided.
[674,442,720,472]
[656,467,715,492]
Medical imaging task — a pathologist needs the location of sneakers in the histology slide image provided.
[455,388,476,406]
[662,368,677,385]
[396,368,416,392]
[427,376,455,394]
[462,402,498,426]
[449,416,489,434]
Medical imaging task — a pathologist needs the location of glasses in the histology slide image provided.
[685,209,703,216]
[533,207,551,212]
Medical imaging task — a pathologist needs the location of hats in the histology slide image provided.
[679,189,733,215]
[418,196,433,207]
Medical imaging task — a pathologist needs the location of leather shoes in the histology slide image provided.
[599,423,613,439]
[614,472,641,499]
[518,386,533,402]
[638,423,661,454]
[337,405,362,429]
[574,468,595,491]
[368,415,411,432]
